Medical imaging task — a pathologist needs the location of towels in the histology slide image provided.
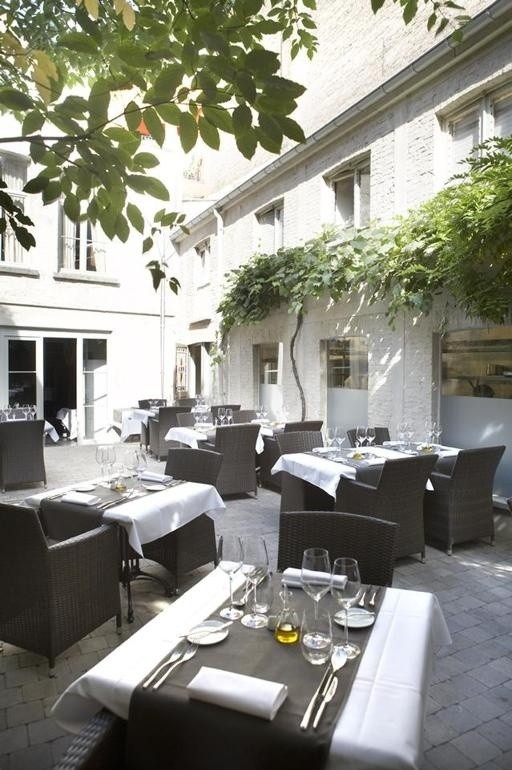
[434,450,458,458]
[280,567,348,595]
[187,666,290,720]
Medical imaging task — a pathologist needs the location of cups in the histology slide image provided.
[299,607,334,665]
[243,566,274,614]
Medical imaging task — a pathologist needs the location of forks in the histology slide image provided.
[142,638,201,691]
[334,612,376,620]
[180,620,233,637]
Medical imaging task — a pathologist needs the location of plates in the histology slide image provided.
[74,482,97,493]
[334,606,375,629]
[186,619,230,645]
[142,482,166,492]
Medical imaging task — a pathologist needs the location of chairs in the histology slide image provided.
[424,445,506,557]
[0,504,121,679]
[335,454,439,563]
[142,448,224,595]
[0,398,391,511]
[277,512,398,588]
[54,706,129,769]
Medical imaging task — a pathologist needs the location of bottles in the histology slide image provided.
[352,441,364,460]
[274,591,300,645]
[115,467,127,491]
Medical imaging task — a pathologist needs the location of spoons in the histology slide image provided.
[358,583,381,608]
[231,576,264,607]
[319,647,349,697]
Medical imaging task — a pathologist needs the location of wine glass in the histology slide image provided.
[147,399,159,410]
[1,401,38,422]
[323,425,347,457]
[192,406,233,430]
[300,546,332,619]
[194,394,212,406]
[355,424,377,454]
[95,443,116,481]
[396,420,415,448]
[328,557,362,660]
[215,533,270,629]
[255,404,269,424]
[124,449,147,497]
[423,419,443,452]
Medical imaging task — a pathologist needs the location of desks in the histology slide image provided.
[164,420,286,454]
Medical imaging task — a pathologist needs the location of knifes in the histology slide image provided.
[95,494,127,510]
[165,479,183,487]
[311,676,339,730]
[47,489,71,501]
